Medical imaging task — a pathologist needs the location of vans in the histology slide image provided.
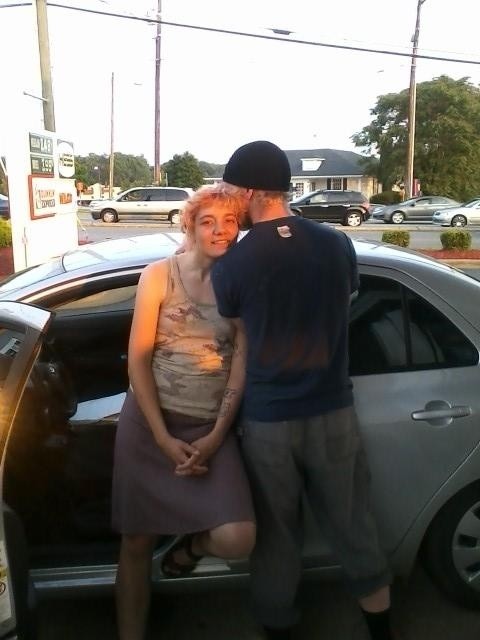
[87,186,197,226]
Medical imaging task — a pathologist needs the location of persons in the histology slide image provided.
[211,140,397,640]
[108,184,261,639]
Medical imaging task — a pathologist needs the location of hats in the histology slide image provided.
[223,141,291,192]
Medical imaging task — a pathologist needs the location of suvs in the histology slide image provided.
[289,189,373,228]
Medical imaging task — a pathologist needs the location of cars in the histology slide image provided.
[0,223,480,640]
[369,194,460,224]
[430,197,480,229]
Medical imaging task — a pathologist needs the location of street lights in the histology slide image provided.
[93,165,101,198]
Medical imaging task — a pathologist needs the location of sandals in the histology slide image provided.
[162,538,201,578]
